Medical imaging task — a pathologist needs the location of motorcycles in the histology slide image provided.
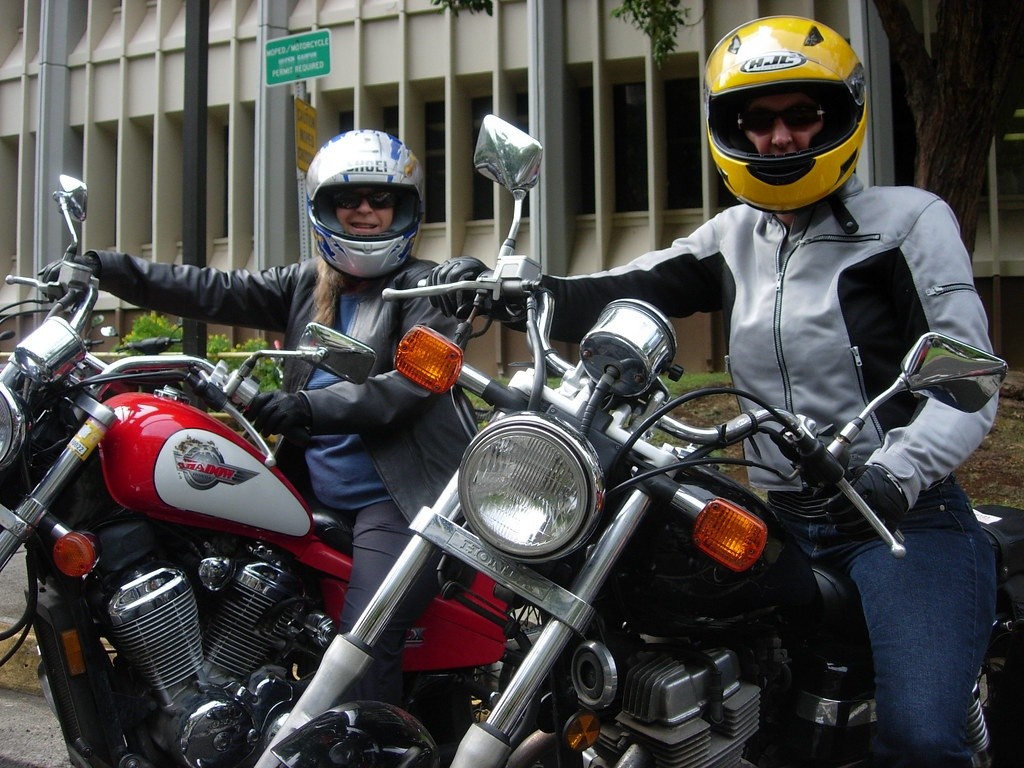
[0,111,1024,768]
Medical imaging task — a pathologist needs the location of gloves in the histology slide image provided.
[38,254,100,283]
[428,257,503,321]
[243,389,311,439]
[825,463,909,542]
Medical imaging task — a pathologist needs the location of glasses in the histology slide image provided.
[737,106,824,132]
[335,191,396,208]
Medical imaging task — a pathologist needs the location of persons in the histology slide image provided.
[429,14,1000,768]
[35,128,480,709]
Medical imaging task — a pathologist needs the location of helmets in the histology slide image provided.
[703,15,867,213]
[305,130,426,279]
